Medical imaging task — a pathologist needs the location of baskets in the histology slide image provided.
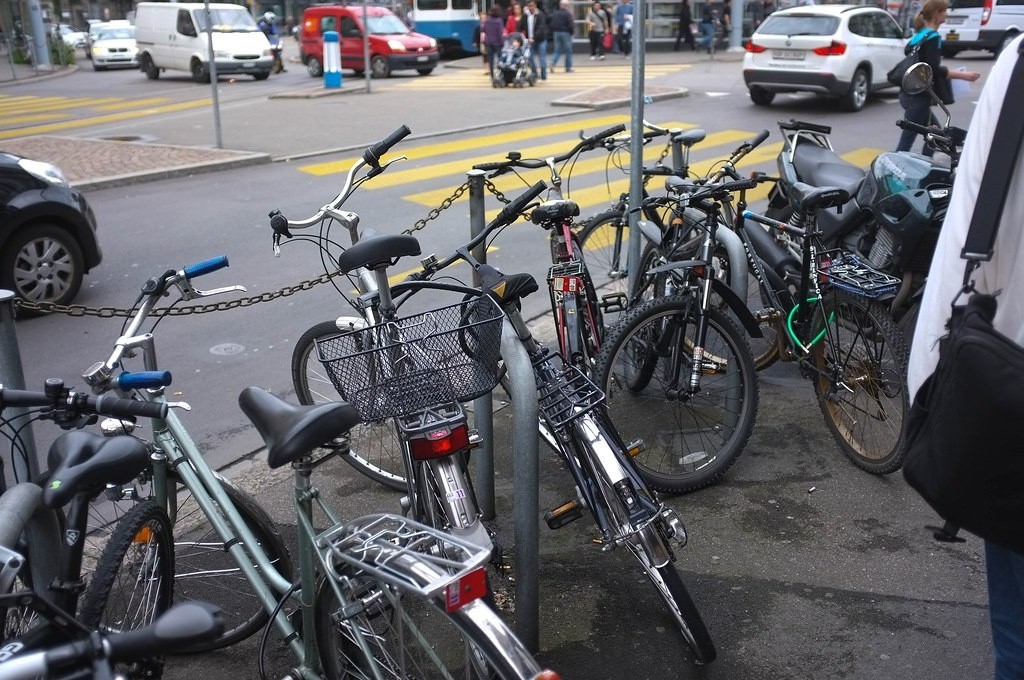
[312,294,504,421]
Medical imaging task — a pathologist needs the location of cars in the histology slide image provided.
[0,147,108,319]
[0,10,143,72]
[743,3,915,112]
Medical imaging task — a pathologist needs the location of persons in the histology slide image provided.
[718,0,731,48]
[257,11,288,72]
[478,0,548,80]
[614,0,633,56]
[589,0,610,61]
[896,0,980,156]
[906,32,1024,680]
[752,0,776,30]
[675,0,696,51]
[549,0,575,73]
[695,0,718,53]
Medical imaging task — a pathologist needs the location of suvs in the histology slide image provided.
[934,0,1023,62]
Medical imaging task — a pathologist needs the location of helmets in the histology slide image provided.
[263,12,277,26]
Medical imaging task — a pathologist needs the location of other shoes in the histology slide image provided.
[565,68,574,72]
[550,65,554,74]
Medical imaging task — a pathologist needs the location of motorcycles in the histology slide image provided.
[759,60,967,357]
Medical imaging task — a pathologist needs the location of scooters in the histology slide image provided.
[265,33,283,74]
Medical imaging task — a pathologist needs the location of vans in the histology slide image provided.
[300,3,440,80]
[134,1,276,84]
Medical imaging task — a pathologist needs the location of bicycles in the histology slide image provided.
[0,114,911,680]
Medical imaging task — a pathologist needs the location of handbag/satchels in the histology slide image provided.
[902,293,1024,556]
[603,33,613,48]
[887,30,935,85]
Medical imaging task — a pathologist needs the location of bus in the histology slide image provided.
[413,0,560,56]
